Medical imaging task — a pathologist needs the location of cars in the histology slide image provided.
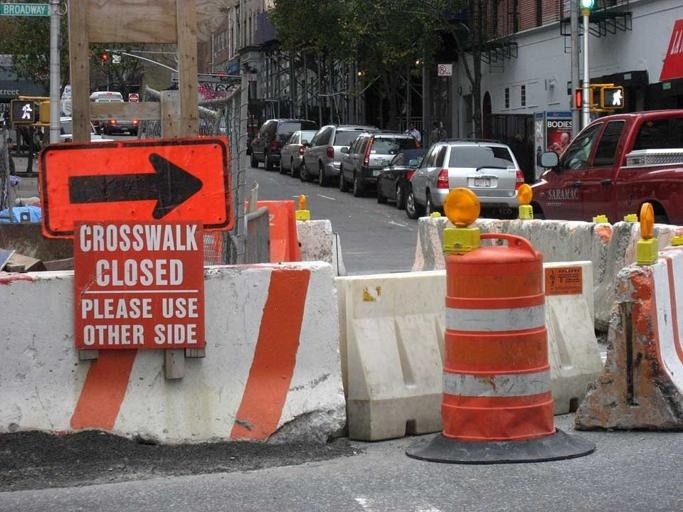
[376,149,428,209]
[89,91,138,135]
[405,139,524,218]
[298,122,381,186]
[278,130,319,177]
[338,131,422,197]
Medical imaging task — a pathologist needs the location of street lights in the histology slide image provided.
[578,0,594,157]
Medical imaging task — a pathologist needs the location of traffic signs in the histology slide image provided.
[3,4,50,16]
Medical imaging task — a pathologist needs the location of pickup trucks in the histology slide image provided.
[530,108,683,225]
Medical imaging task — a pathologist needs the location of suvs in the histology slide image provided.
[249,118,319,170]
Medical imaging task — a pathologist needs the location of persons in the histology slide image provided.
[427,121,448,149]
[404,122,422,150]
[547,131,571,151]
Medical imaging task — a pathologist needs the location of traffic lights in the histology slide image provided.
[599,86,625,110]
[10,99,35,124]
[575,88,582,111]
[98,52,110,64]
